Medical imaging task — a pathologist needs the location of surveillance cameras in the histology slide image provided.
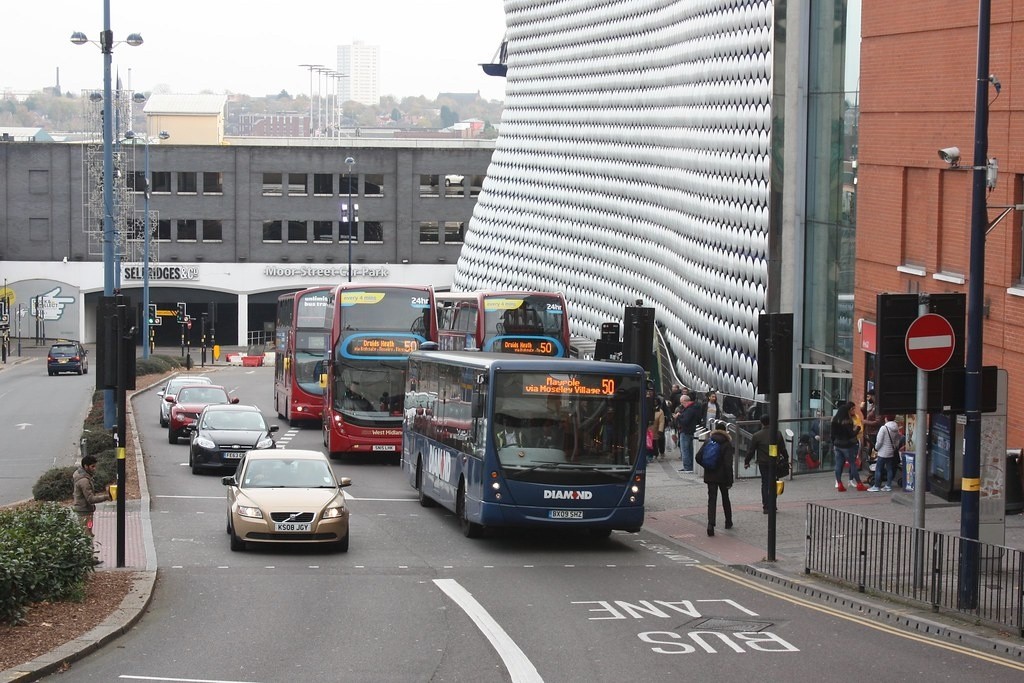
[938,146,960,163]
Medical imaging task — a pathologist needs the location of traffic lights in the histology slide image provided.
[177,302,190,323]
[0,302,9,322]
[148,304,162,325]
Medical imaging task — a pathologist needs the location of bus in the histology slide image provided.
[435,291,573,356]
[273,286,348,427]
[319,283,442,459]
[400,349,655,543]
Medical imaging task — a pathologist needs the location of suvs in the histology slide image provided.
[48,341,89,376]
[157,374,240,445]
[189,403,280,474]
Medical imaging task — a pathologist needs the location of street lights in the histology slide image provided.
[345,157,355,284]
[70,29,144,433]
[124,127,170,359]
[299,63,350,145]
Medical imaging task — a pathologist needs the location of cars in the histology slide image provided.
[429,174,465,187]
[221,449,351,553]
[269,176,382,244]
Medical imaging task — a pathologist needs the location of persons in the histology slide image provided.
[646,384,720,472]
[495,416,531,450]
[743,414,789,514]
[72,455,111,538]
[695,423,734,536]
[809,389,900,492]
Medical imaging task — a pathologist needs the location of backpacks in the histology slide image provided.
[702,437,723,469]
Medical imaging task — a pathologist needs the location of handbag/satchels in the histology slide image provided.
[804,446,821,470]
[646,427,653,449]
[776,453,790,478]
[893,449,901,464]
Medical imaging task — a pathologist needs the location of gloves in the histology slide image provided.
[856,426,861,431]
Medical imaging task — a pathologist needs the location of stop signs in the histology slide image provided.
[905,312,956,371]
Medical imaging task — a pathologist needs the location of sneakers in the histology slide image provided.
[880,485,892,491]
[867,485,879,492]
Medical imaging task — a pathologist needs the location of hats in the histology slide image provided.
[865,389,875,395]
[886,414,896,421]
[800,433,809,442]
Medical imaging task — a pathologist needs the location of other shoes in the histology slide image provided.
[725,522,733,529]
[857,481,868,491]
[835,480,838,488]
[837,480,847,492]
[849,479,857,487]
[763,509,768,514]
[659,453,665,459]
[707,525,714,536]
[678,468,694,473]
[94,560,104,565]
